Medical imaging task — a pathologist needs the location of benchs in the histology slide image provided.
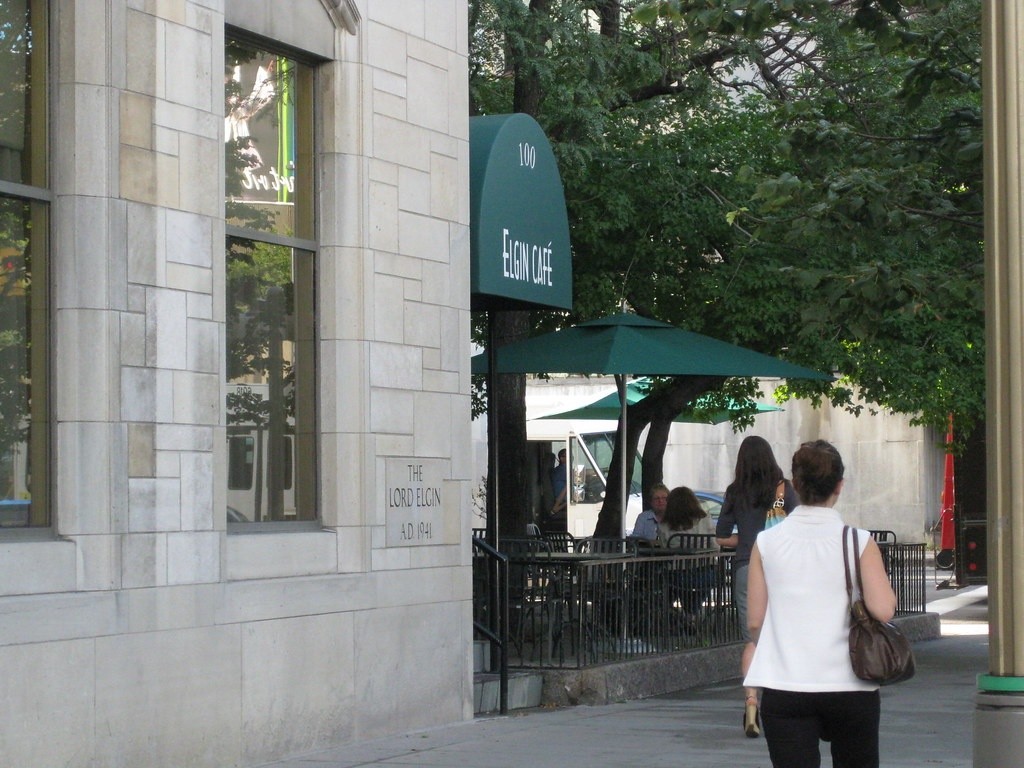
[666,533,725,629]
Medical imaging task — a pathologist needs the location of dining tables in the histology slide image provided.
[516,552,635,651]
[633,546,718,635]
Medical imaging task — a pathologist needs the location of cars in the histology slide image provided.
[692,490,739,529]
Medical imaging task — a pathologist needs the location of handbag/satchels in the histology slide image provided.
[764,480,787,531]
[842,525,915,685]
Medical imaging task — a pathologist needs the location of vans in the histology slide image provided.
[526,419,671,552]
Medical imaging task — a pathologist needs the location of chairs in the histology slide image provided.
[867,530,896,580]
[571,538,638,648]
[546,531,593,605]
[498,538,579,665]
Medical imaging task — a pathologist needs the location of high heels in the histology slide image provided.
[743,695,760,738]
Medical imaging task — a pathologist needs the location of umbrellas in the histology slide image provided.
[470,299,844,643]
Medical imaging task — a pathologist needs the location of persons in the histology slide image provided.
[552,448,575,501]
[747,440,898,768]
[716,436,794,737]
[628,483,721,623]
